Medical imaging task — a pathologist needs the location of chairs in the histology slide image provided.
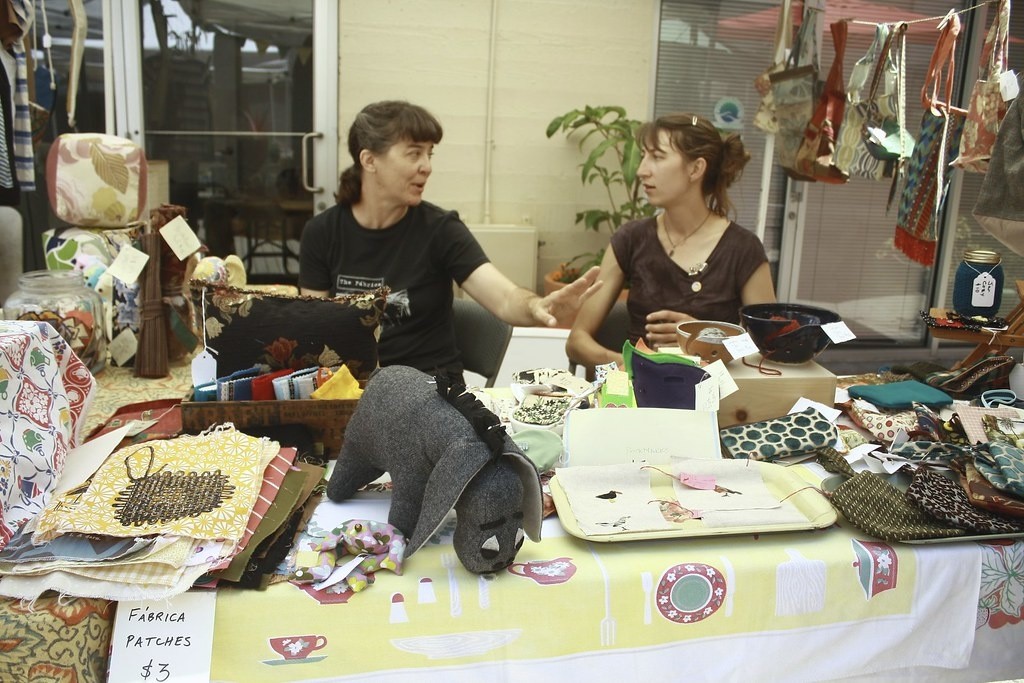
[452,299,513,388]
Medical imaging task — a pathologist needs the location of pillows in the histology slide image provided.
[186,278,392,379]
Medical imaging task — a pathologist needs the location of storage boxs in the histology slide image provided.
[182,379,366,459]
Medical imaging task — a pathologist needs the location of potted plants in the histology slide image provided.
[541,102,656,329]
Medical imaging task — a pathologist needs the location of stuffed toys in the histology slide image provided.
[326,364,544,575]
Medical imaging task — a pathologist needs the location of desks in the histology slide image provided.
[216,197,312,279]
[0,353,192,683]
[103,374,1024,683]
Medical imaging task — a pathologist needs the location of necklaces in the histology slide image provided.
[663,211,712,257]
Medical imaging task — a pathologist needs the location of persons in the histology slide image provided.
[565,112,776,372]
[298,100,604,388]
